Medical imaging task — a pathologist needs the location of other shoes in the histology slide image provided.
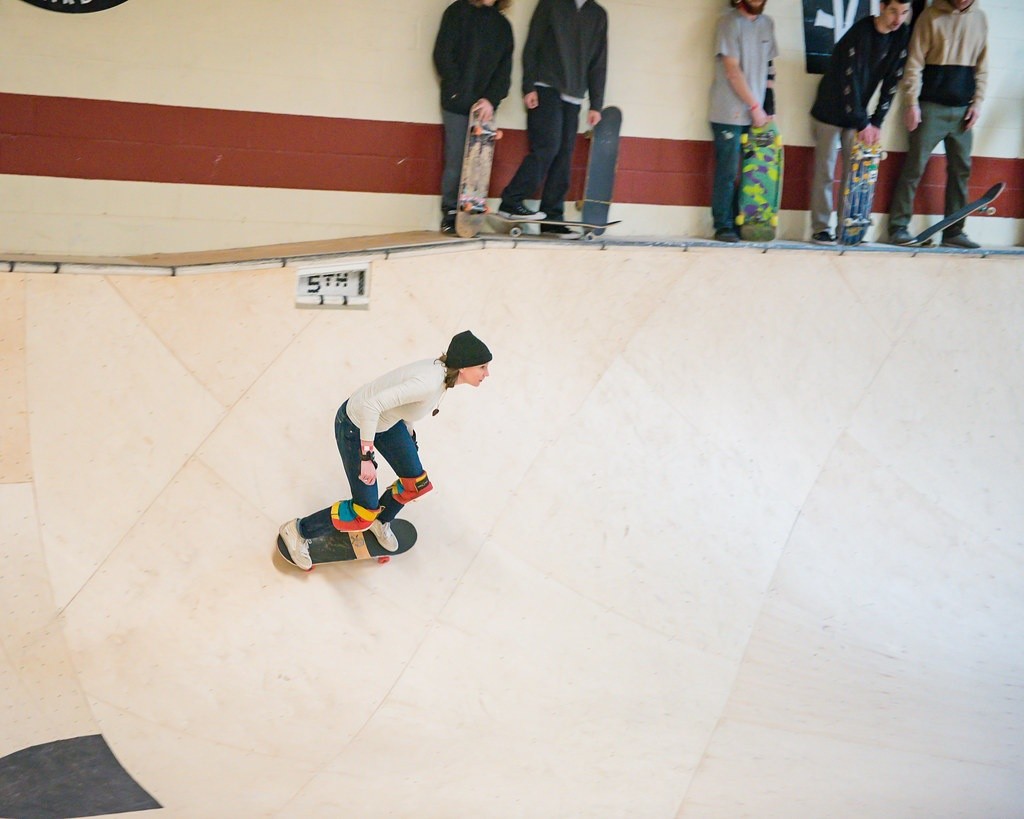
[715,228,738,242]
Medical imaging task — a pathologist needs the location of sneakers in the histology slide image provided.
[498,201,547,221]
[811,232,836,246]
[942,232,980,249]
[541,225,581,240]
[886,229,918,246]
[369,519,399,552]
[279,518,312,571]
[442,219,482,238]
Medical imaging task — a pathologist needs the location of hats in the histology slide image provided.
[446,331,492,368]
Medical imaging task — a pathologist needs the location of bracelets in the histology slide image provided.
[749,102,760,111]
[361,445,374,451]
[359,448,378,469]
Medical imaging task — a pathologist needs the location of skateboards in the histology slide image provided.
[575,106,622,237]
[486,211,622,241]
[836,126,888,248]
[455,101,503,238]
[277,518,418,572]
[734,117,783,241]
[904,182,1005,248]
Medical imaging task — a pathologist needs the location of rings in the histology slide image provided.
[365,479,367,482]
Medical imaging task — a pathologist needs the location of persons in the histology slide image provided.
[279,330,493,571]
[432,0,515,237]
[495,0,607,238]
[706,0,778,243]
[809,0,910,245]
[887,0,982,249]
